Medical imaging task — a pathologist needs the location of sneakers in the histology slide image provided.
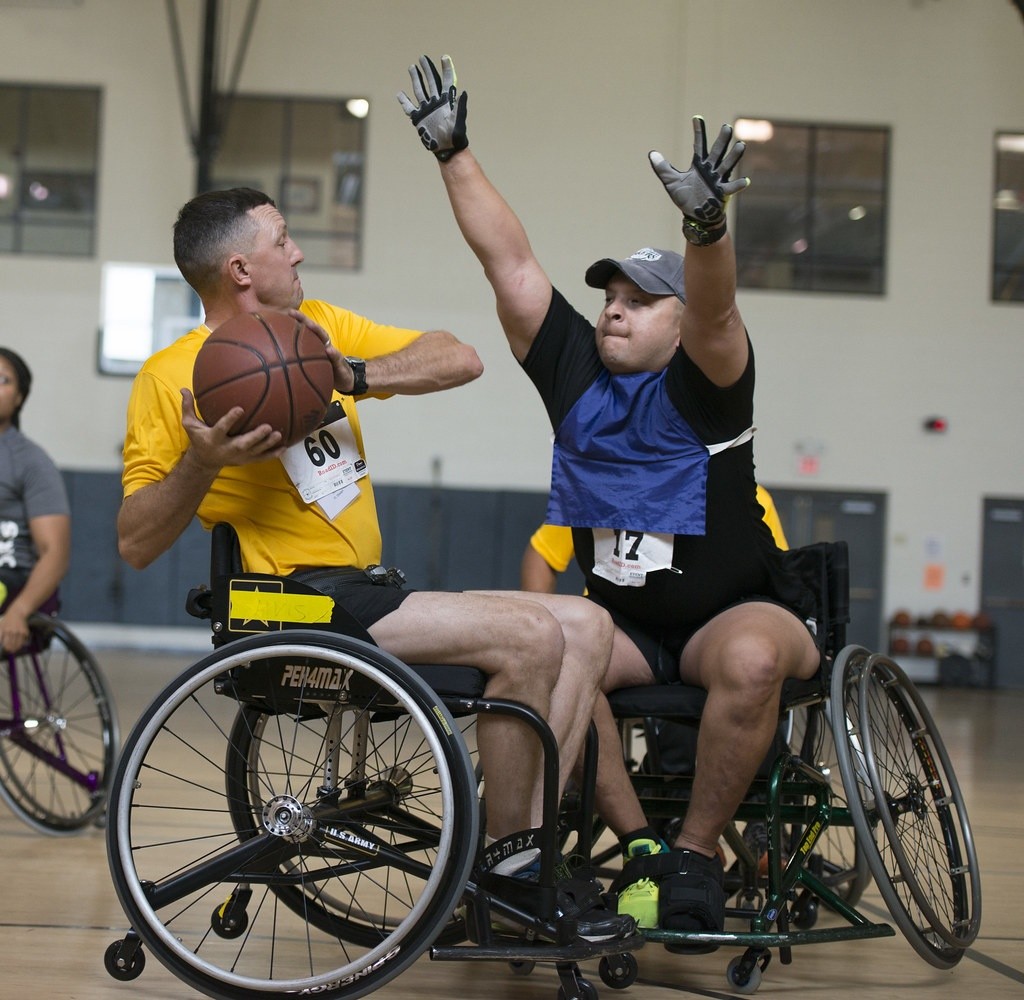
[665,844,727,955]
[453,856,640,949]
[617,839,669,930]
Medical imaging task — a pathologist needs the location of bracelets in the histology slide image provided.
[336,355,368,397]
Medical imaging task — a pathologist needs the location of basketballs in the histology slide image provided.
[192,309,335,447]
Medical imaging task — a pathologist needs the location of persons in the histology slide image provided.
[506,481,796,596]
[395,52,837,957]
[115,184,637,949]
[0,345,74,662]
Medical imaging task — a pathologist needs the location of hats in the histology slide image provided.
[585,248,686,306]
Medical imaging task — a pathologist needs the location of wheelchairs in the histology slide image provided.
[0,610,114,834]
[105,521,644,1000]
[462,539,983,994]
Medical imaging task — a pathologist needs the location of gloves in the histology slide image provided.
[397,54,469,163]
[648,114,750,231]
